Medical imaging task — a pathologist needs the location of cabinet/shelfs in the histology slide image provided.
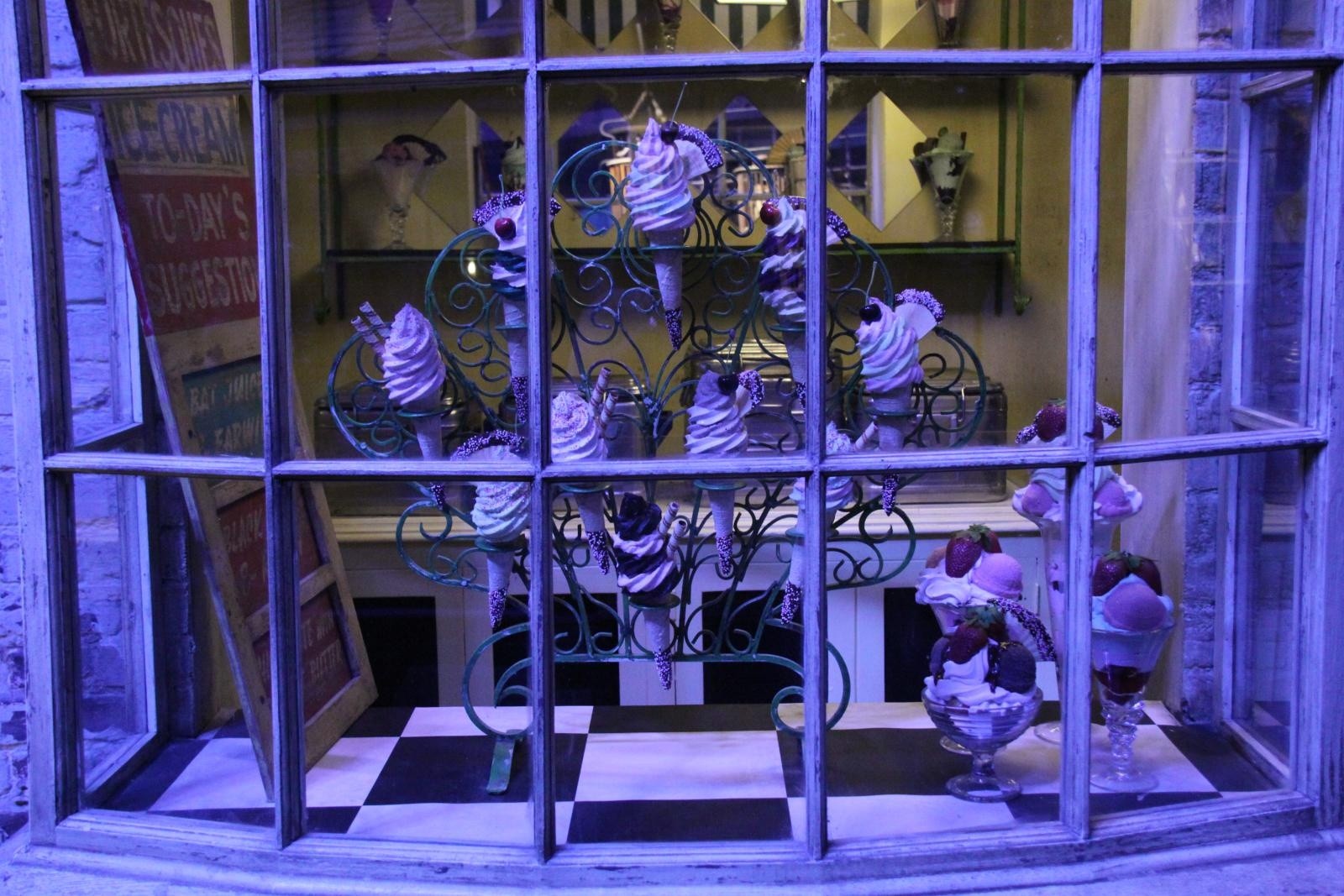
[310,1,1034,320]
[340,567,468,710]
[848,561,1039,710]
[464,562,673,708]
[666,561,857,708]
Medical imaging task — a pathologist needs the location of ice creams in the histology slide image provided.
[347,109,1179,742]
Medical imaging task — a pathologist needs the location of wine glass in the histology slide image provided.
[1090,623,1176,791]
[921,148,973,242]
[370,157,423,249]
[923,683,1044,802]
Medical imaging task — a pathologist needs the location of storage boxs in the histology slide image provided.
[679,337,843,509]
[317,391,483,517]
[843,368,1006,505]
[497,374,674,511]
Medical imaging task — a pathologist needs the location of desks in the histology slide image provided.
[328,475,1057,710]
